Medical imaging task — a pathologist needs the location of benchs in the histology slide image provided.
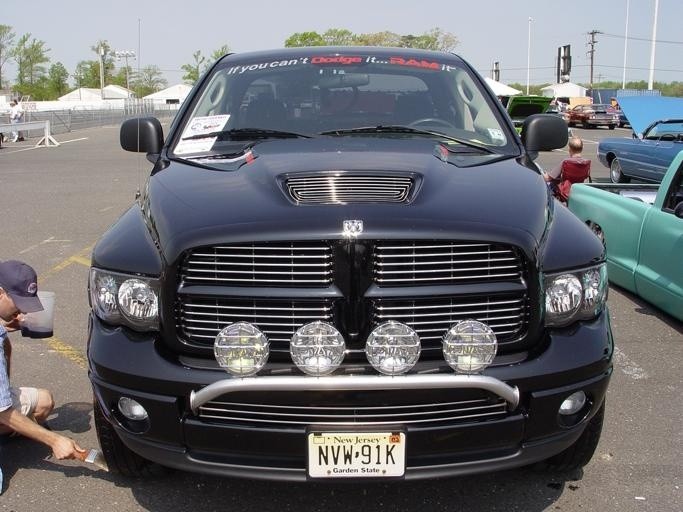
[317,94,395,116]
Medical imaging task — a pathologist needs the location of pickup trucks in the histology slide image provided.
[565,147,683,323]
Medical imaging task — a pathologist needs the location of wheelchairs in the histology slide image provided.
[541,167,592,207]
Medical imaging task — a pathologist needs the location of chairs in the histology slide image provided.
[246,99,288,129]
[393,93,434,126]
[550,161,592,208]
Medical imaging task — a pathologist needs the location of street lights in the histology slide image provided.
[526,16,537,95]
[95,41,105,100]
[114,50,137,95]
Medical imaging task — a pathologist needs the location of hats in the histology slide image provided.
[0,261,43,312]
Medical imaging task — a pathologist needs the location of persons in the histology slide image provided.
[543,137,589,207]
[0,259,86,500]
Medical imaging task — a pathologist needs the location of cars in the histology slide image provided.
[595,95,683,184]
[496,86,662,138]
[84,42,613,503]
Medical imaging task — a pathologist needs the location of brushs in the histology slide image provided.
[80,448,109,472]
